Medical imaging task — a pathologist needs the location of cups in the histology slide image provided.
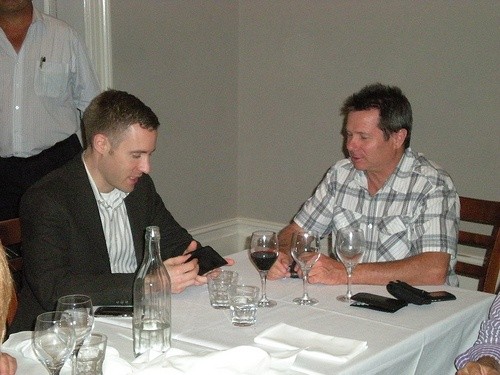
[70,332,107,375]
[228,285,260,326]
[206,271,240,308]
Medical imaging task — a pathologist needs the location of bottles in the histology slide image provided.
[132,225,172,358]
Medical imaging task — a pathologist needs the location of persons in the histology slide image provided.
[266,83,460,287]
[0,241,17,375]
[11,89,234,332]
[454,291,500,375]
[0,0,100,222]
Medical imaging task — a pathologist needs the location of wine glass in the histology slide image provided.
[55,294,94,375]
[32,311,76,375]
[334,227,366,303]
[248,230,279,308]
[291,230,322,306]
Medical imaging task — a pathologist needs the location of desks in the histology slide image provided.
[0,249,496,375]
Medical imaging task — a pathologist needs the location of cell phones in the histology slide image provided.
[95,306,133,318]
[351,293,406,313]
[429,291,456,302]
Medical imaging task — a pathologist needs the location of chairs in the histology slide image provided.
[455,196,500,295]
[0,218,24,289]
